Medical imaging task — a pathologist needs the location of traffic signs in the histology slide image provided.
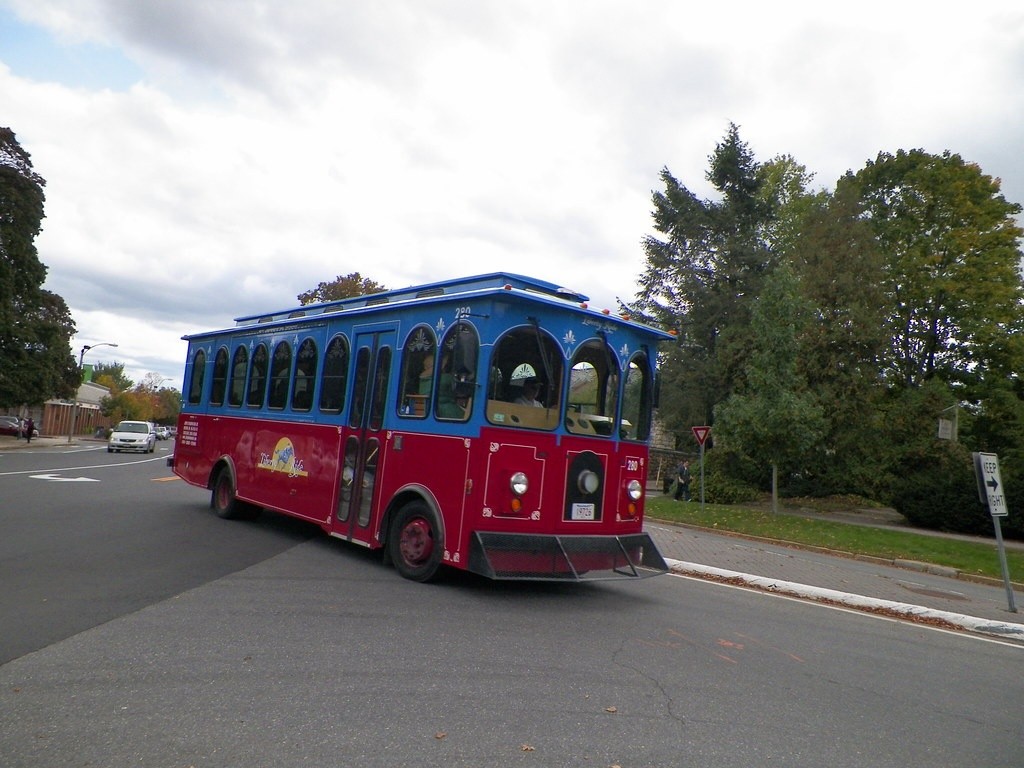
[980,452,1009,516]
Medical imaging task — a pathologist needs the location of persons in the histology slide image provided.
[419,355,463,418]
[251,379,309,409]
[663,463,674,494]
[513,377,544,408]
[17,419,24,440]
[673,460,693,502]
[26,418,34,443]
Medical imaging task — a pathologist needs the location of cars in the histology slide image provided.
[0,416,19,424]
[107,421,157,452]
[152,426,177,441]
[0,419,40,439]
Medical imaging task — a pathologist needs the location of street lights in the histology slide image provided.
[67,343,119,443]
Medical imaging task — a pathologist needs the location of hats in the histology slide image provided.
[420,355,448,378]
[524,377,544,385]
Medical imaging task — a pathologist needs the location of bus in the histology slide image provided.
[167,272,670,589]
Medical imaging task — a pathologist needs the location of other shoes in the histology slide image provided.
[687,498,692,502]
[673,499,677,501]
